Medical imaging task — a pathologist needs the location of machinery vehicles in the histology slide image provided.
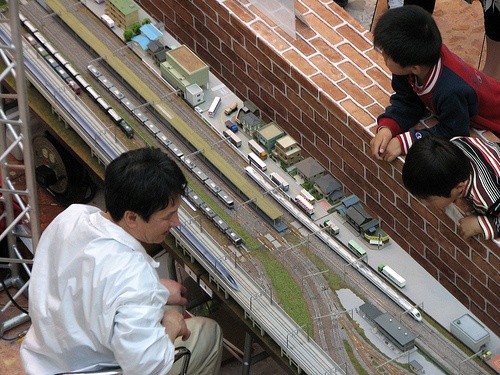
[224,102,238,115]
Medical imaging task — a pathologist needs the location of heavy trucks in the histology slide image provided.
[296,194,315,216]
[378,264,406,288]
[249,139,266,160]
[270,172,289,191]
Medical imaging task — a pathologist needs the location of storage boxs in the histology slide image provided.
[5,105,48,161]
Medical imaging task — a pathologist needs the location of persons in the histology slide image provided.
[369,4,500,162]
[332,0,500,85]
[401,136,500,242]
[17,147,224,375]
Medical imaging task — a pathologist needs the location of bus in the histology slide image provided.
[300,188,315,205]
[249,152,267,172]
[348,240,366,257]
[208,96,221,117]
[223,129,242,147]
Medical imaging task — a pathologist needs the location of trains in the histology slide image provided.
[181,182,241,247]
[175,221,239,291]
[18,11,134,139]
[88,65,234,209]
[246,165,422,323]
[24,32,81,94]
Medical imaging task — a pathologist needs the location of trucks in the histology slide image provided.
[225,120,239,133]
[324,220,339,235]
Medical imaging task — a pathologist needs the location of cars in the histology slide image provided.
[195,106,203,114]
